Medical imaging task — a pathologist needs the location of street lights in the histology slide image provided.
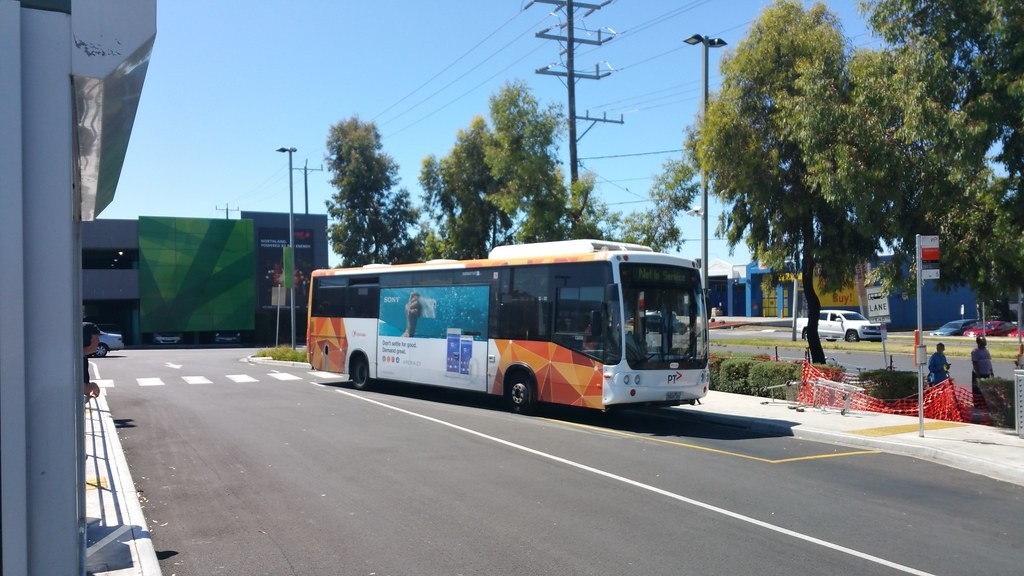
[275,146,296,351]
[683,32,726,311]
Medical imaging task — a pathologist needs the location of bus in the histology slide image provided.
[306,239,711,416]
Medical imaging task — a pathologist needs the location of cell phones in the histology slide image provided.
[417,296,436,318]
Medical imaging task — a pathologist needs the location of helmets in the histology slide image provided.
[83,322,101,347]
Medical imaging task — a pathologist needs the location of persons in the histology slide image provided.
[82,321,101,397]
[928,342,950,384]
[971,334,994,407]
[399,292,422,338]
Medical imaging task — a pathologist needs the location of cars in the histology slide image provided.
[214,329,243,345]
[152,331,185,345]
[642,308,688,336]
[88,330,125,358]
[962,320,1024,337]
[929,318,979,336]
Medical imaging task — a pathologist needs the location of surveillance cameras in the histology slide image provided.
[687,206,702,216]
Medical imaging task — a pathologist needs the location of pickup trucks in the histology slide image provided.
[792,309,882,343]
